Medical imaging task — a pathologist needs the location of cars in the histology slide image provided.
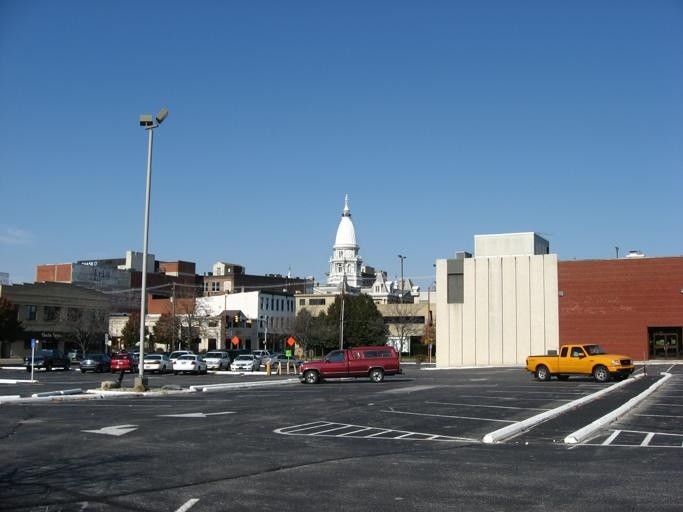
[78,347,305,376]
[67,349,82,360]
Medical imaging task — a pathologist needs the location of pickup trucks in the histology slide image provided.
[525,343,634,383]
[298,346,402,385]
[23,348,70,372]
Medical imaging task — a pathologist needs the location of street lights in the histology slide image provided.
[398,254,406,303]
[222,289,230,351]
[131,107,167,389]
